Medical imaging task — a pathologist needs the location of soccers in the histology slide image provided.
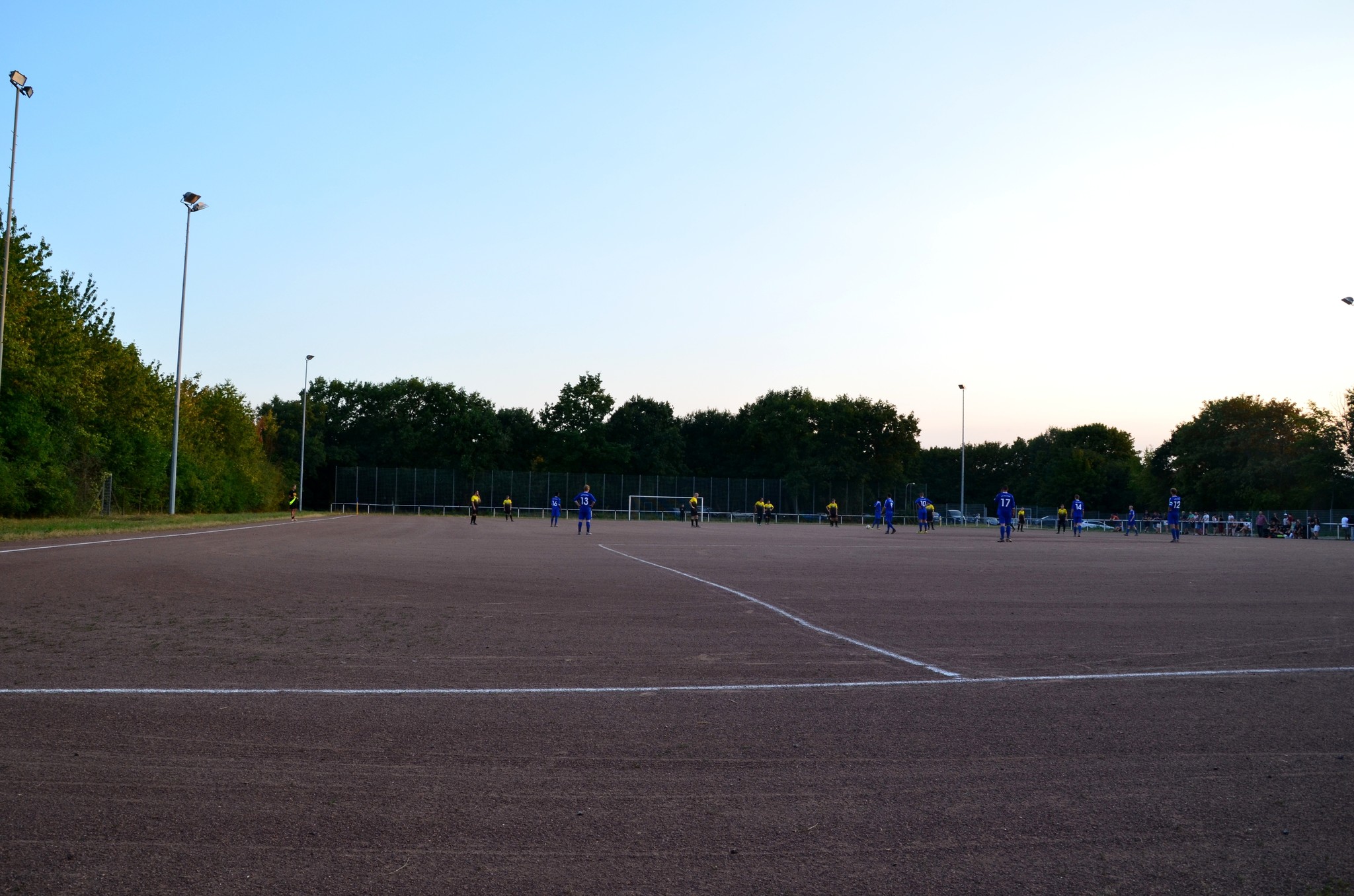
[866,525,871,530]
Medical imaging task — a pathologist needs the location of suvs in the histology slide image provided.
[945,510,966,523]
[697,506,721,518]
[1080,520,1115,530]
[1031,515,1069,525]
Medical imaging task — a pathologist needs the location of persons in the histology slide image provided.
[915,491,932,533]
[763,500,774,524]
[503,495,514,522]
[754,497,765,526]
[1179,510,1354,541]
[880,492,896,534]
[470,490,481,524]
[680,503,686,521]
[550,492,562,527]
[994,484,1026,543]
[1110,510,1169,534]
[1071,495,1084,537]
[1056,504,1068,534]
[288,484,300,521]
[689,492,702,527]
[826,498,839,527]
[925,502,935,530]
[574,484,596,535]
[1124,505,1139,536]
[1167,488,1180,543]
[871,498,882,530]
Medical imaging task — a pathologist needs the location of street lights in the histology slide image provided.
[905,483,916,513]
[958,385,966,525]
[169,191,209,514]
[0,70,34,373]
[299,355,315,512]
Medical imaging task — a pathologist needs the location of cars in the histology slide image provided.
[964,515,979,523]
[987,517,1000,525]
[817,513,827,517]
[727,509,757,522]
[668,506,688,516]
[932,512,941,522]
[858,513,871,519]
[797,511,827,523]
